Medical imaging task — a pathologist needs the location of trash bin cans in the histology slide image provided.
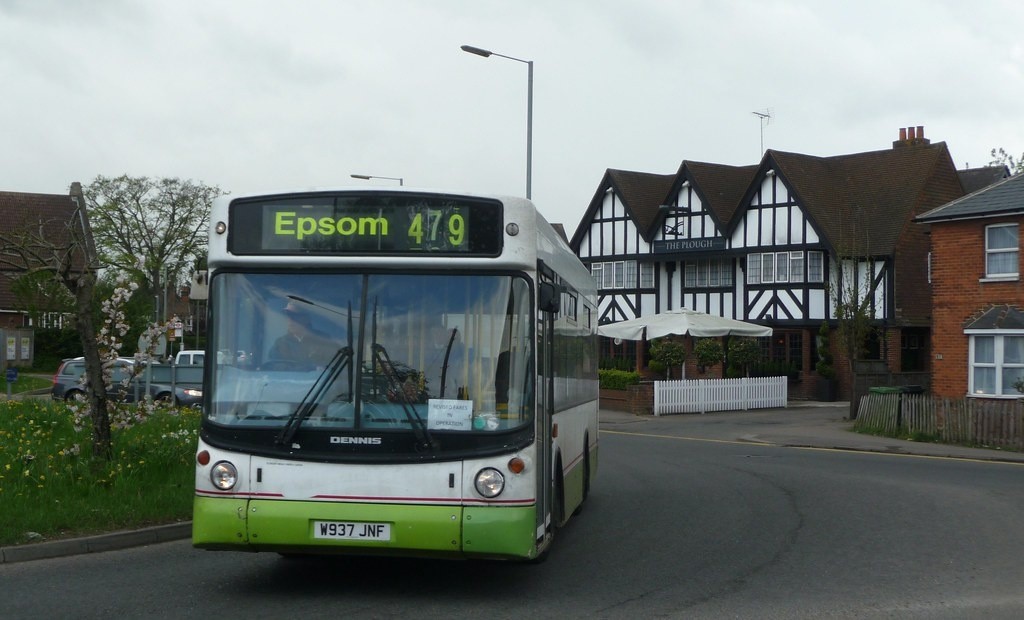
[847,358,931,421]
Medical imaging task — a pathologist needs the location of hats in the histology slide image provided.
[282,302,309,314]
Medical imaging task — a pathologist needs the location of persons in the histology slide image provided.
[256,300,340,371]
[446,328,473,367]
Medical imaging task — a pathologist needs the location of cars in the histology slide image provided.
[234,350,251,366]
[124,363,207,406]
[53,359,137,404]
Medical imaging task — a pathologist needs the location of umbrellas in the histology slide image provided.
[598,307,774,379]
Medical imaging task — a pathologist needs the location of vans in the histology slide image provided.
[175,350,225,368]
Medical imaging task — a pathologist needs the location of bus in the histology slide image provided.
[191,184,601,573]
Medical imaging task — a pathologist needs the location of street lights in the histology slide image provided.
[350,173,405,189]
[460,43,534,199]
